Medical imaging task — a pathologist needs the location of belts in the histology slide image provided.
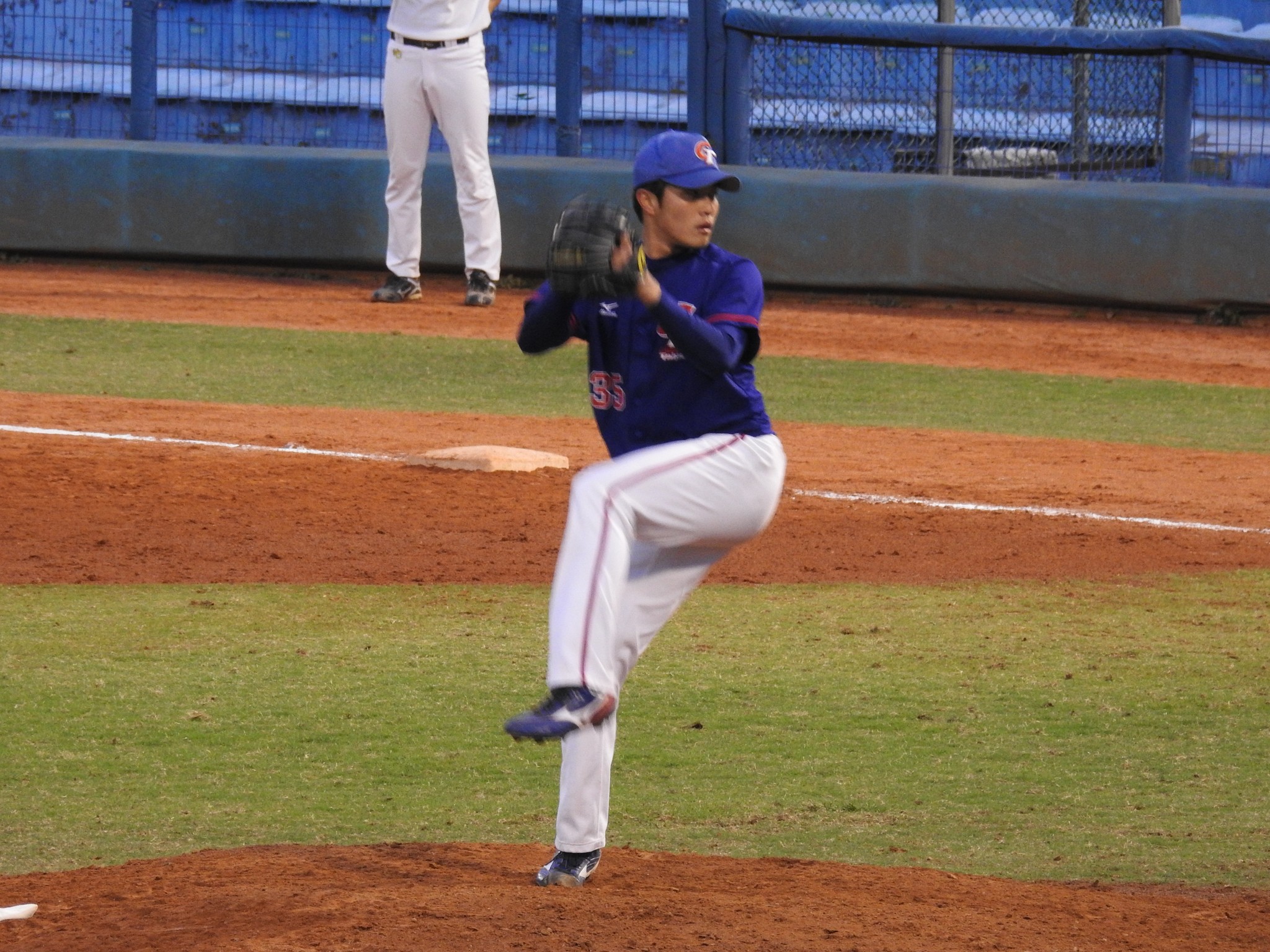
[391,33,471,48]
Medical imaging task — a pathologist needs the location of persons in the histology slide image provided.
[373,0,501,305]
[499,129,786,888]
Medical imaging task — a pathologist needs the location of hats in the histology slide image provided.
[632,130,741,195]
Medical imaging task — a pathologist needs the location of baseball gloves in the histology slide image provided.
[547,192,647,307]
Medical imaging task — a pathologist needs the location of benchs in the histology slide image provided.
[0,0,1269,192]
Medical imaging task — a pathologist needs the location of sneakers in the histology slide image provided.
[373,279,422,300]
[502,687,617,744]
[462,270,495,307]
[536,849,603,889]
